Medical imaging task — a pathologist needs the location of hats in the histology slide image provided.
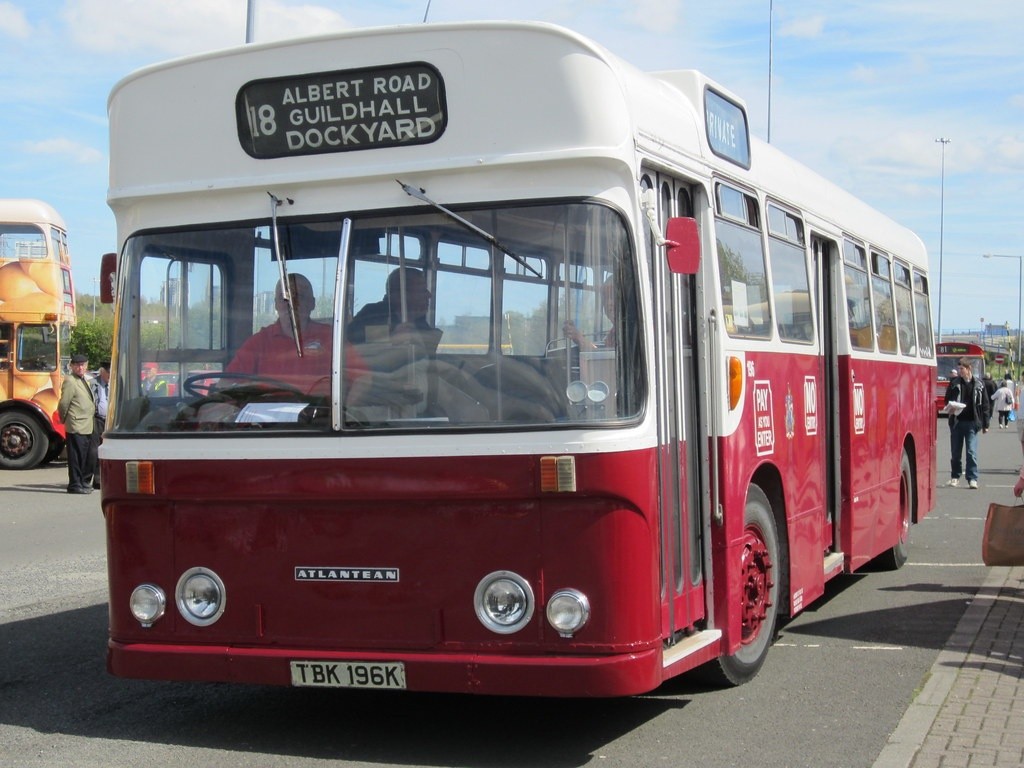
[71,355,88,364]
[100,362,111,371]
[143,361,157,369]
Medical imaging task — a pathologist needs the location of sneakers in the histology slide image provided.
[950,478,959,488]
[968,479,976,489]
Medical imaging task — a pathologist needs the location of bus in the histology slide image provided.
[937,342,988,415]
[1,197,77,469]
[99,21,937,701]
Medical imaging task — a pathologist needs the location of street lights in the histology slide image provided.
[984,254,1022,380]
[935,138,951,344]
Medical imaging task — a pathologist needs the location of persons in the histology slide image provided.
[57,351,96,498]
[215,269,371,420]
[942,357,992,488]
[344,264,440,367]
[564,273,622,365]
[88,361,109,488]
[946,367,1016,436]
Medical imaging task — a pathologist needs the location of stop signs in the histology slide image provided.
[995,354,1004,364]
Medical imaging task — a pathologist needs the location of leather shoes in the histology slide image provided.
[68,484,95,495]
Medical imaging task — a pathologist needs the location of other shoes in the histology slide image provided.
[1000,423,1008,428]
